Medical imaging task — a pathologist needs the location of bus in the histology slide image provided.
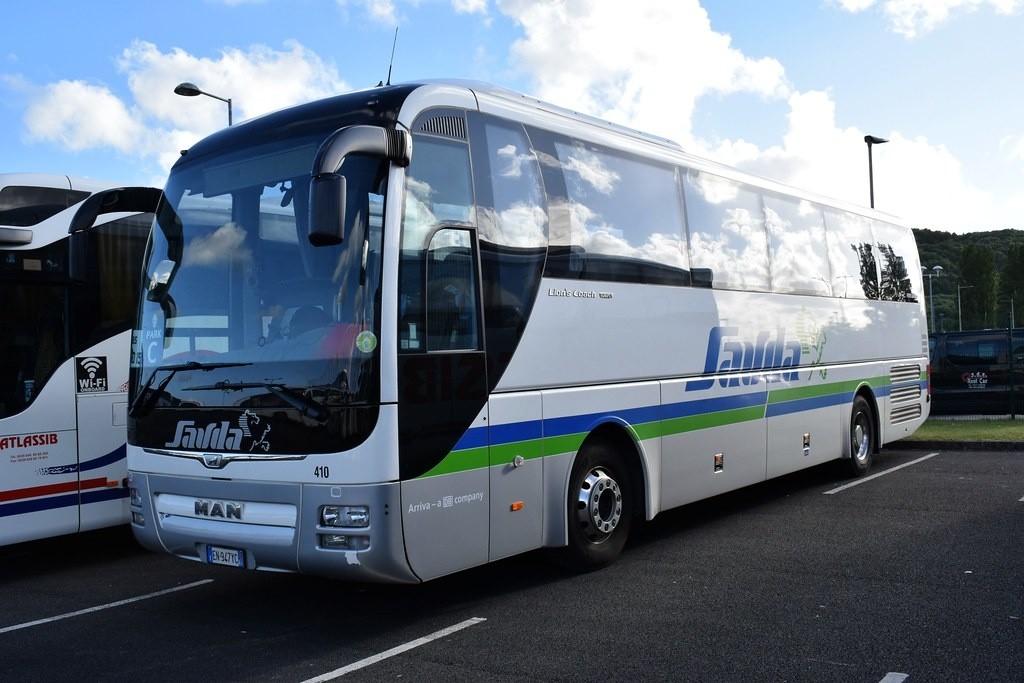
[0,178,174,550]
[64,27,931,593]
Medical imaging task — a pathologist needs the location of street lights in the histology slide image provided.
[863,134,890,210]
[920,265,943,335]
[957,284,976,332]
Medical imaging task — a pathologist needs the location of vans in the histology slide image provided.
[925,328,1024,387]
